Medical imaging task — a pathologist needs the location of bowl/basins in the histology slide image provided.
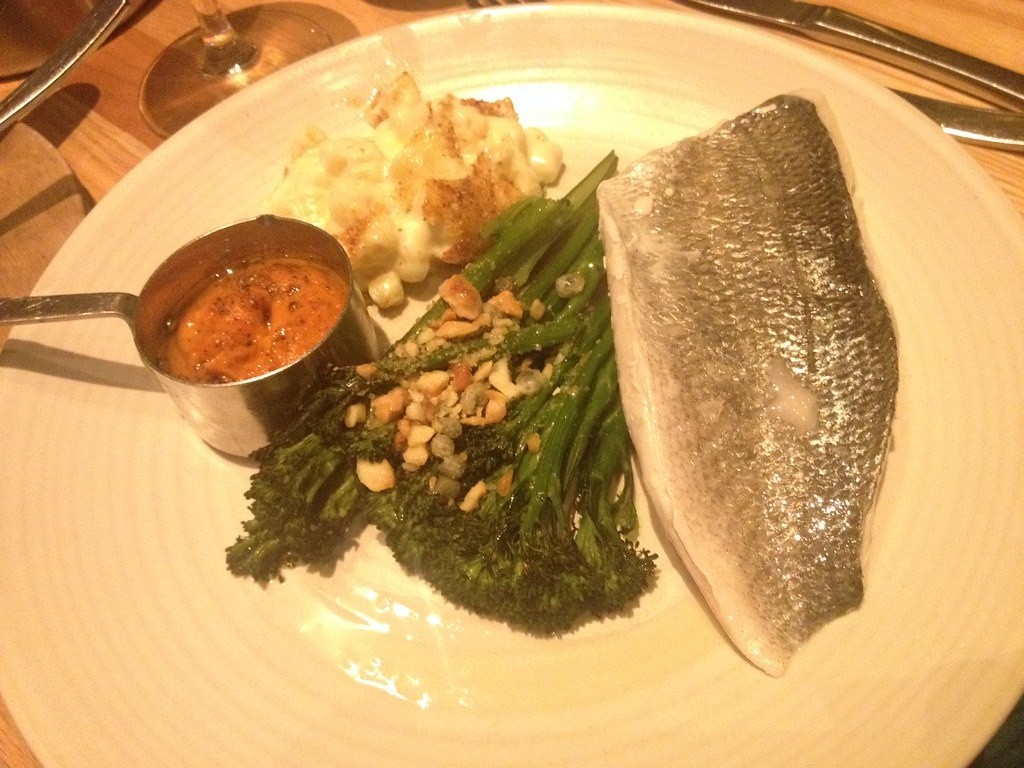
[0,215,378,456]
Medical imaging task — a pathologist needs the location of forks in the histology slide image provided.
[467,0,543,8]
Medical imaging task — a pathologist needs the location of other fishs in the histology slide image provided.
[597,92,901,679]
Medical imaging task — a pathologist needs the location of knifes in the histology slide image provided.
[692,0,1024,113]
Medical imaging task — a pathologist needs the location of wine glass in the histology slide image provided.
[138,0,360,140]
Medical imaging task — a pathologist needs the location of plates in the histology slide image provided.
[1,3,1024,768]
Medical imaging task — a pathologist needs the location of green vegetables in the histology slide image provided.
[226,150,661,636]
